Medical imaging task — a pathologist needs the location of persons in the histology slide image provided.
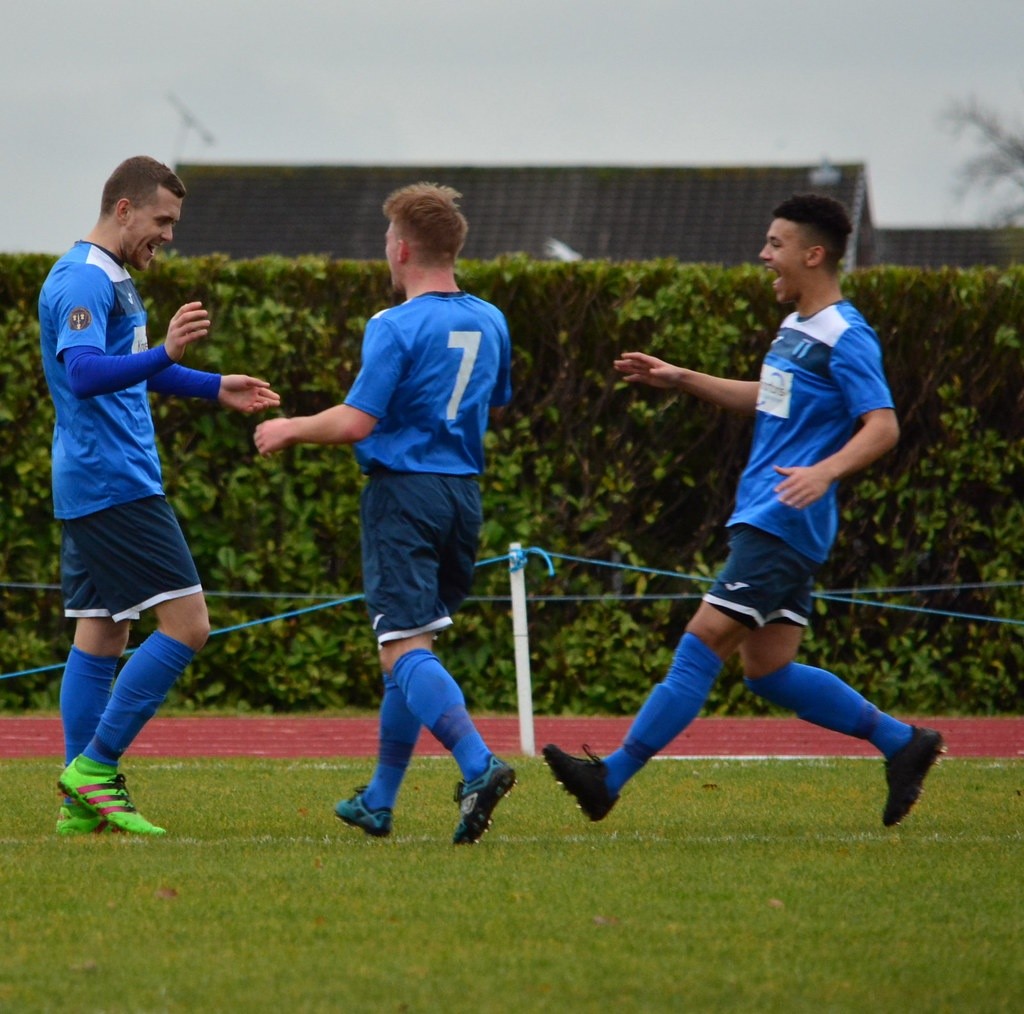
[541,194,945,826]
[250,183,520,847]
[36,157,280,837]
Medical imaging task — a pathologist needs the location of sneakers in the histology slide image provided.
[57,757,166,836]
[883,724,948,826]
[335,786,392,836]
[543,743,620,821]
[451,754,518,844]
[56,792,128,834]
